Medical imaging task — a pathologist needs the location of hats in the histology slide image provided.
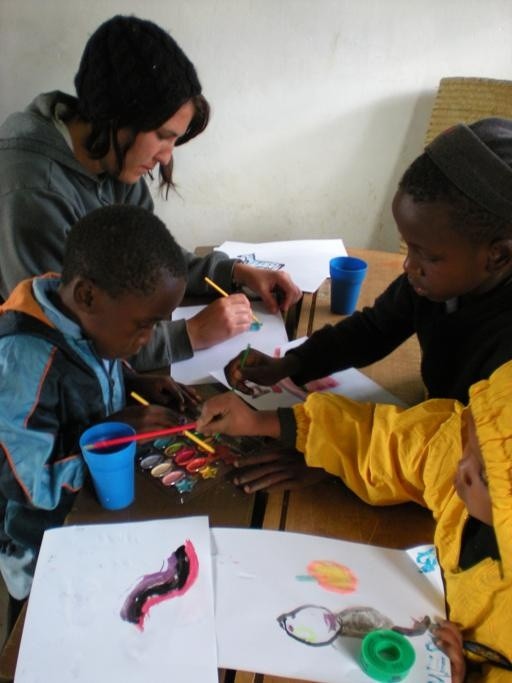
[75,16,201,120]
[422,117,512,220]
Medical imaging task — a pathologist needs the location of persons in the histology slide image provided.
[0,203,187,601]
[224,119,512,493]
[196,360,511,683]
[0,14,303,378]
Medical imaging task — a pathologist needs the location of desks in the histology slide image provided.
[236,249,437,681]
[1,245,290,682]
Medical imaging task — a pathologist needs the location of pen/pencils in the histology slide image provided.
[82,391,216,454]
[232,344,251,391]
[203,275,262,326]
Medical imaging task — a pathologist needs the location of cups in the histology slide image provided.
[328,256,368,315]
[76,422,138,513]
